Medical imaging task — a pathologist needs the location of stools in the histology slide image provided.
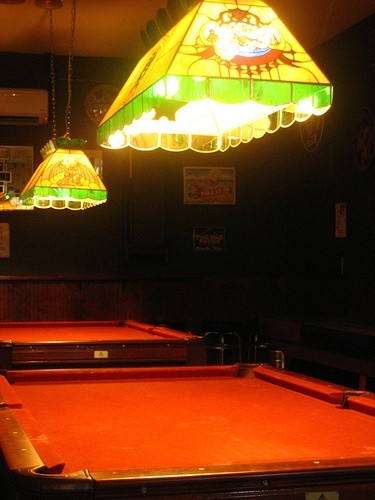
[203,330,274,365]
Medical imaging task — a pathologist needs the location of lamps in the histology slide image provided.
[99,0,335,153]
[18,0,108,210]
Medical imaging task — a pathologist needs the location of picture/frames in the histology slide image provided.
[193,226,226,251]
[183,165,236,205]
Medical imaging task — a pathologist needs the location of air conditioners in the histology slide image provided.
[0,88,49,126]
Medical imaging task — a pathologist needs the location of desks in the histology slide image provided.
[0,362,375,500]
[0,319,205,370]
